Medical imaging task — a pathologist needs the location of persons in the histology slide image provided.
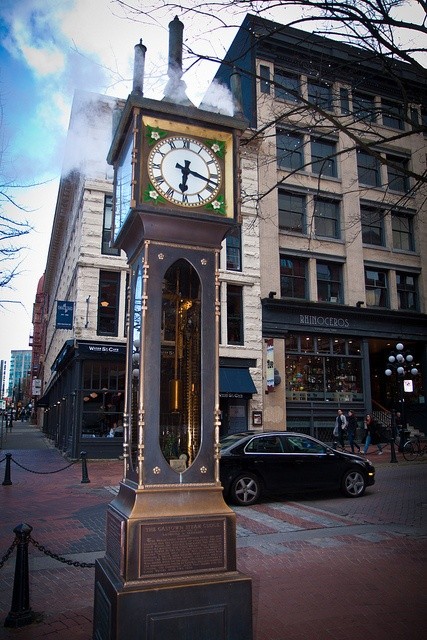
[347,410,362,453]
[108,420,118,438]
[395,411,404,436]
[116,421,123,435]
[361,414,383,455]
[331,407,347,448]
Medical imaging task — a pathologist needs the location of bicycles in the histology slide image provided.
[403,434,427,461]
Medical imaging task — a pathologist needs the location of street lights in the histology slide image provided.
[385,341,418,450]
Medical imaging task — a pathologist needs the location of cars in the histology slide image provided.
[220,431,375,506]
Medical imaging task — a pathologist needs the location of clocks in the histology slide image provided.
[136,117,239,222]
